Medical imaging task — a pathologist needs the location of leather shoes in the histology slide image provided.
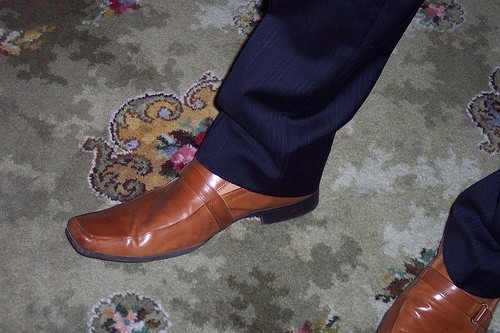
[373,233,500,333]
[65,157,319,264]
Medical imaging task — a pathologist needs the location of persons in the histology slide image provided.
[65,0,500,333]
